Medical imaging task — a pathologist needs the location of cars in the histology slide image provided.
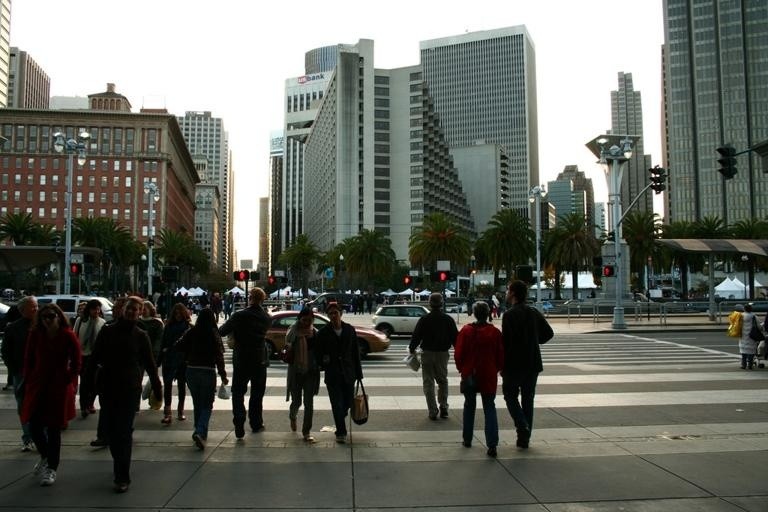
[229,309,391,357]
[445,302,468,312]
[373,304,432,336]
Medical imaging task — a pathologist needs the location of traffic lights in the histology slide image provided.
[603,263,615,277]
[440,272,446,280]
[268,276,289,284]
[604,265,614,277]
[268,277,277,284]
[251,272,260,282]
[234,270,260,281]
[70,262,82,274]
[430,271,457,282]
[70,264,82,276]
[404,277,410,284]
[402,277,411,286]
[232,270,248,281]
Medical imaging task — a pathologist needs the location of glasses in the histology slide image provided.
[40,313,58,320]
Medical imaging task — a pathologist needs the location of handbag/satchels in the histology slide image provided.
[352,379,370,426]
[749,317,766,342]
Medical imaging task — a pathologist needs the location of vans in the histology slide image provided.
[30,295,116,325]
[307,293,348,311]
[632,293,652,303]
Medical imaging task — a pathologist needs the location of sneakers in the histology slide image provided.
[21,439,36,452]
[252,423,265,433]
[161,415,173,424]
[290,417,298,431]
[335,433,348,444]
[33,461,48,476]
[40,468,57,486]
[178,414,186,420]
[234,416,246,439]
[113,482,129,493]
[90,440,108,447]
[462,440,473,448]
[303,433,314,441]
[487,447,497,457]
[192,433,206,449]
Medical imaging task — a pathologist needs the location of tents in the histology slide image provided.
[270,285,317,298]
[380,287,457,301]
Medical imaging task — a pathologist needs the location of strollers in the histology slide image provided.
[752,323,768,368]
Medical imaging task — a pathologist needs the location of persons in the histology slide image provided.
[496,294,503,318]
[89,284,246,324]
[2,290,36,391]
[738,304,761,370]
[408,293,460,421]
[90,296,148,447]
[19,303,82,486]
[465,293,476,316]
[160,303,194,424]
[0,296,38,452]
[285,308,321,441]
[454,300,502,458]
[173,308,229,451]
[136,300,165,412]
[487,295,498,322]
[492,294,499,318]
[73,299,107,419]
[218,287,272,440]
[78,301,89,316]
[499,279,544,449]
[85,296,163,494]
[312,303,363,443]
[269,290,407,315]
